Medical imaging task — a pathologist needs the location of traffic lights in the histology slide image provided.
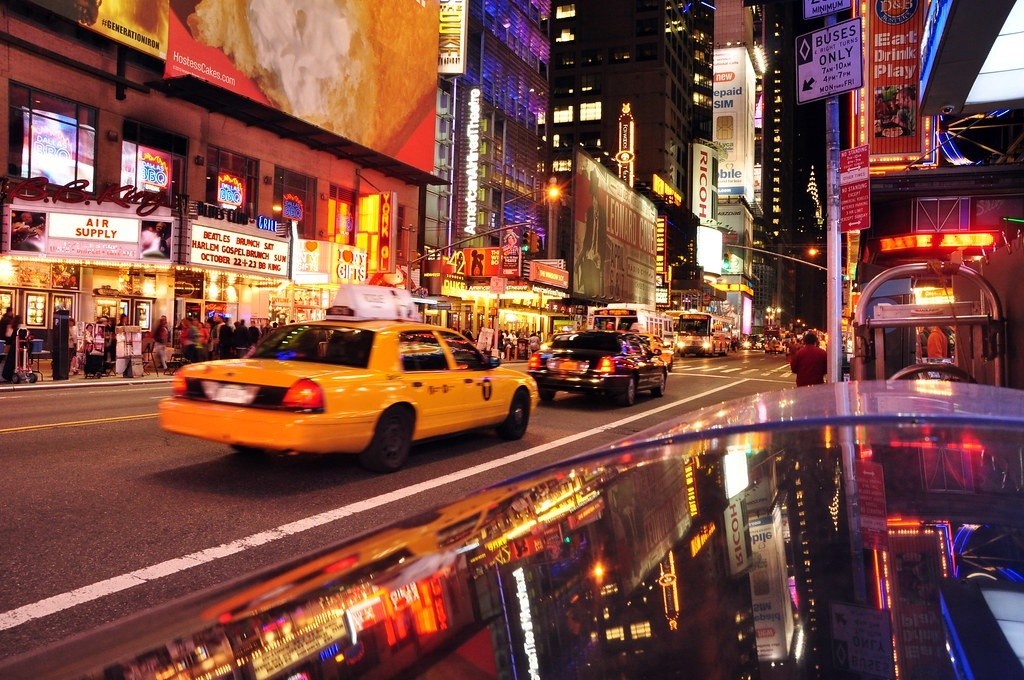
[722,252,731,270]
[808,248,817,256]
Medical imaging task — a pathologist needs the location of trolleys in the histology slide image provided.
[82,341,105,379]
[12,328,39,384]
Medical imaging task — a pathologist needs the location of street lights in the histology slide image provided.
[498,183,564,307]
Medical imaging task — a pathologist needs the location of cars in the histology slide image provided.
[527,328,668,408]
[763,329,801,355]
[539,330,583,352]
[637,333,673,373]
[157,281,540,476]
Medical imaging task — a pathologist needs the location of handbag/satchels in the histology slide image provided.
[501,352,505,359]
[73,342,77,351]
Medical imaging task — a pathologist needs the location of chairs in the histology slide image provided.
[162,342,193,376]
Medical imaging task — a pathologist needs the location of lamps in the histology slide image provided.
[107,130,118,141]
[263,176,273,185]
[195,154,204,166]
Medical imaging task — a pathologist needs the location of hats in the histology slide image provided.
[100,318,107,322]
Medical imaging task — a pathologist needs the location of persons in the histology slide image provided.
[502,232,520,256]
[916,326,956,358]
[574,170,606,298]
[156,222,170,258]
[732,332,827,354]
[0,307,32,384]
[68,314,169,375]
[790,334,827,387]
[874,86,916,137]
[178,313,295,363]
[21,212,44,227]
[450,326,554,360]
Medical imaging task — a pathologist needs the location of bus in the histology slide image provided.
[676,308,733,356]
[591,302,676,360]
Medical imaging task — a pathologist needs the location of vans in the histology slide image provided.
[742,334,761,351]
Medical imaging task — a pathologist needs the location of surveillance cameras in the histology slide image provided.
[941,105,954,115]
